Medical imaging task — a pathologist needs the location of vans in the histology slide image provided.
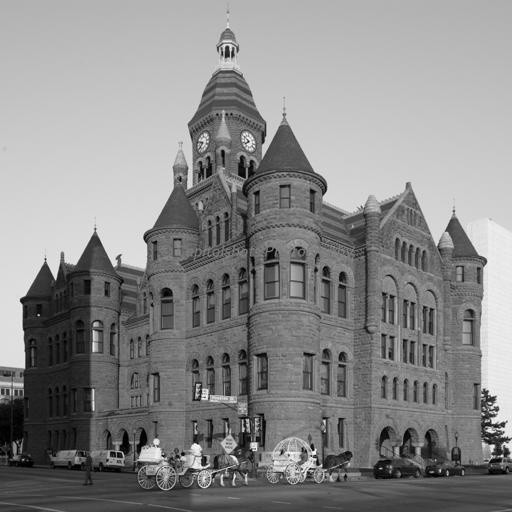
[373,458,422,479]
[86,449,126,471]
[51,448,93,470]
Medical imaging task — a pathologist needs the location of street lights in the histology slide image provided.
[454,430,458,465]
[132,427,137,471]
[319,419,326,466]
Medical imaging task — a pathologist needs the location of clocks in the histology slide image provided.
[197,126,258,156]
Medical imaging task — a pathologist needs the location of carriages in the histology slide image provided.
[265,435,353,485]
[136,436,257,490]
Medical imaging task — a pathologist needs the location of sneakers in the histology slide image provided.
[83,483,93,486]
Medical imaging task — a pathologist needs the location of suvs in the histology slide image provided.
[487,457,512,474]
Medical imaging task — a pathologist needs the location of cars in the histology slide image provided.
[9,454,35,467]
[484,458,490,464]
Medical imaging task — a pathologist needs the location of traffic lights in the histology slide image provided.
[254,417,259,433]
[193,381,202,401]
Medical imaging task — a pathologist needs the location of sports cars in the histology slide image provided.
[425,460,466,477]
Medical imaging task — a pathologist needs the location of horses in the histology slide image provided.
[212,448,255,487]
[324,451,353,482]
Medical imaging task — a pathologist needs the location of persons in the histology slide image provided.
[83,451,94,486]
[152,434,208,473]
[278,448,290,461]
[310,443,320,465]
[296,448,308,471]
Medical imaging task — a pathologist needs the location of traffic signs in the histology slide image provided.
[209,395,237,404]
[201,388,209,401]
